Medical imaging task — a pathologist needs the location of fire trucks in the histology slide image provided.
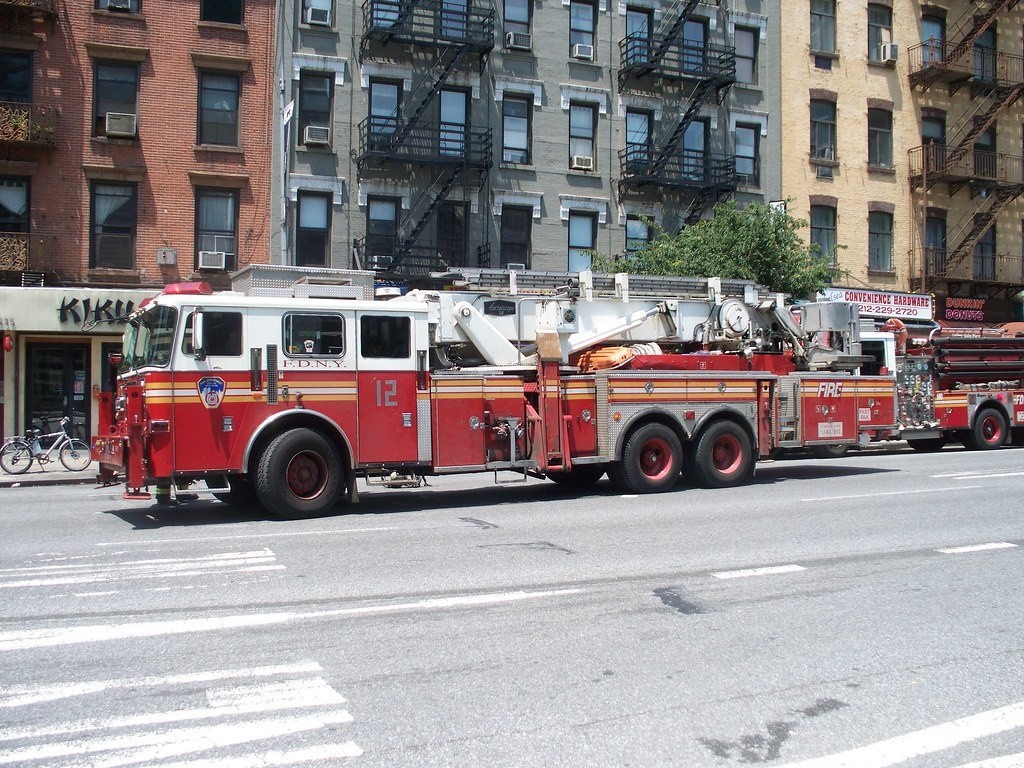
[81,263,907,520]
[898,322,1024,451]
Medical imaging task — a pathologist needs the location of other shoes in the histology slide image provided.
[157,497,178,506]
[175,493,199,502]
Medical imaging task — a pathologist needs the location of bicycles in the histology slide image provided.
[0,417,92,474]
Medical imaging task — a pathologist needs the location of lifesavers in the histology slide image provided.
[879,318,909,351]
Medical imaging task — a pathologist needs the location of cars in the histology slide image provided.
[32,412,85,440]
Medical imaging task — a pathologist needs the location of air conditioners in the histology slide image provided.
[880,43,899,62]
[303,125,330,145]
[570,155,594,171]
[105,112,138,137]
[306,7,331,26]
[504,31,533,49]
[107,0,131,11]
[504,262,527,272]
[196,251,226,271]
[572,44,594,60]
[366,257,392,270]
